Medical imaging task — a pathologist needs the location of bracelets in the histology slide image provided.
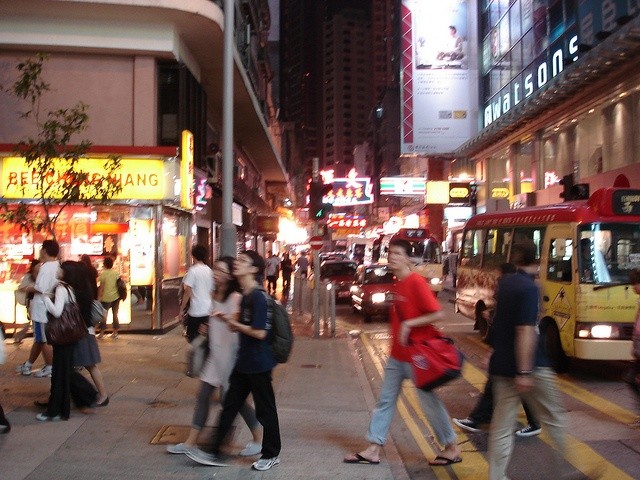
[516,370,532,377]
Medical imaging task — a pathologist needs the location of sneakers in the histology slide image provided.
[35,366,52,377]
[183,447,216,466]
[515,424,542,438]
[452,417,481,433]
[15,361,31,376]
[251,457,280,471]
[240,440,262,456]
[37,413,69,420]
[166,443,198,454]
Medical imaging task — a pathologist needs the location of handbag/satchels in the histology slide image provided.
[90,300,107,326]
[44,304,89,345]
[407,332,464,392]
[117,278,127,300]
[185,346,206,377]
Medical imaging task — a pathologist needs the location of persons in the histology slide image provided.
[446,26,463,52]
[71,262,110,408]
[185,250,282,472]
[36,261,98,424]
[452,262,542,438]
[281,254,292,289]
[629,267,640,398]
[179,243,215,354]
[21,259,41,321]
[167,256,264,457]
[79,252,100,336]
[342,239,463,465]
[15,240,62,379]
[486,238,605,480]
[96,257,124,340]
[263,251,279,294]
[296,250,309,286]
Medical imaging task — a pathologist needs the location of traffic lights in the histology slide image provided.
[311,183,333,220]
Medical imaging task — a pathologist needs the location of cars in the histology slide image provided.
[320,260,357,303]
[350,264,396,321]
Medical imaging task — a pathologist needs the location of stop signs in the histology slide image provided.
[310,236,323,250]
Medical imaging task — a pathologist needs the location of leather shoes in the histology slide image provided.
[34,399,50,406]
[98,396,108,405]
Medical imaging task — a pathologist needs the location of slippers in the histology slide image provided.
[344,453,380,464]
[429,456,463,466]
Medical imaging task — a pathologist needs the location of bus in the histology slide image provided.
[372,228,443,297]
[455,187,640,374]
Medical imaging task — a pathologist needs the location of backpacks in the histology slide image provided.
[272,299,292,363]
